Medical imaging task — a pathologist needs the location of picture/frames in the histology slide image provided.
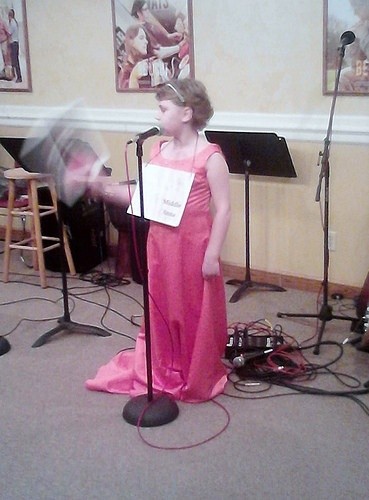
[322,0,369,97]
[111,0,195,94]
[0,0,32,93]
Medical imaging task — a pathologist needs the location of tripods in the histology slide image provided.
[276,48,364,355]
[202,130,299,303]
[0,137,112,347]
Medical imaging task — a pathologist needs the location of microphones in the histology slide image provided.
[337,30,357,49]
[126,123,165,144]
[233,348,274,368]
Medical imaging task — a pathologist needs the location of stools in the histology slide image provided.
[3,167,78,290]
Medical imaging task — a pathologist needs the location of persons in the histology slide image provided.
[336,0,368,94]
[85,80,230,405]
[113,2,190,90]
[0,9,21,82]
